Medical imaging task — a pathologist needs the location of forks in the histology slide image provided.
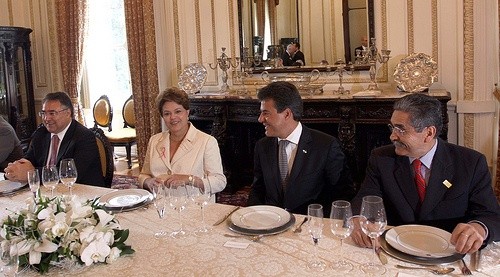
[462,259,472,276]
[294,217,308,235]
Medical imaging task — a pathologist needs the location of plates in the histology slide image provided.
[384,224,457,260]
[0,179,27,194]
[99,189,150,208]
[377,229,466,265]
[394,52,438,92]
[94,193,154,212]
[178,63,207,94]
[226,214,296,235]
[230,206,291,232]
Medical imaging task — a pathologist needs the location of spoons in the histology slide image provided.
[396,264,455,275]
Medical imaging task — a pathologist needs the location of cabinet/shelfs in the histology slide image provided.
[0,26,37,157]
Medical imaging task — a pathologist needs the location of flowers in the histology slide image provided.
[0,189,136,275]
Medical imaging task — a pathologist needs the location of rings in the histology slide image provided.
[461,232,470,238]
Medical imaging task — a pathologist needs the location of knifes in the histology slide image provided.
[213,205,240,227]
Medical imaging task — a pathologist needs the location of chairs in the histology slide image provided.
[105,95,137,169]
[93,95,114,152]
[88,127,114,188]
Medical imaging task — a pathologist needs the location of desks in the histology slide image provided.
[184,88,452,205]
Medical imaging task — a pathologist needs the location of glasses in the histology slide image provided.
[38,109,68,118]
[388,123,425,137]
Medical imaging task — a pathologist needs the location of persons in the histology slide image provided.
[0,118,24,173]
[3,92,107,188]
[355,37,369,63]
[137,86,227,204]
[243,80,355,220]
[346,94,500,256]
[275,41,306,65]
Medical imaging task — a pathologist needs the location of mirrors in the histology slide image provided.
[237,0,375,74]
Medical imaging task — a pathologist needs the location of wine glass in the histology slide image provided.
[168,180,190,238]
[191,178,211,234]
[27,168,40,200]
[152,181,171,240]
[360,196,386,275]
[330,200,354,272]
[42,164,58,198]
[306,203,327,271]
[59,159,77,200]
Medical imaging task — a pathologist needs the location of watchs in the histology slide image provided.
[189,175,194,187]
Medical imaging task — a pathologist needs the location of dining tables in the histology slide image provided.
[0,173,500,277]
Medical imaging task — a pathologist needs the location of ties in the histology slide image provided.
[48,134,60,171]
[277,139,292,200]
[411,158,427,206]
